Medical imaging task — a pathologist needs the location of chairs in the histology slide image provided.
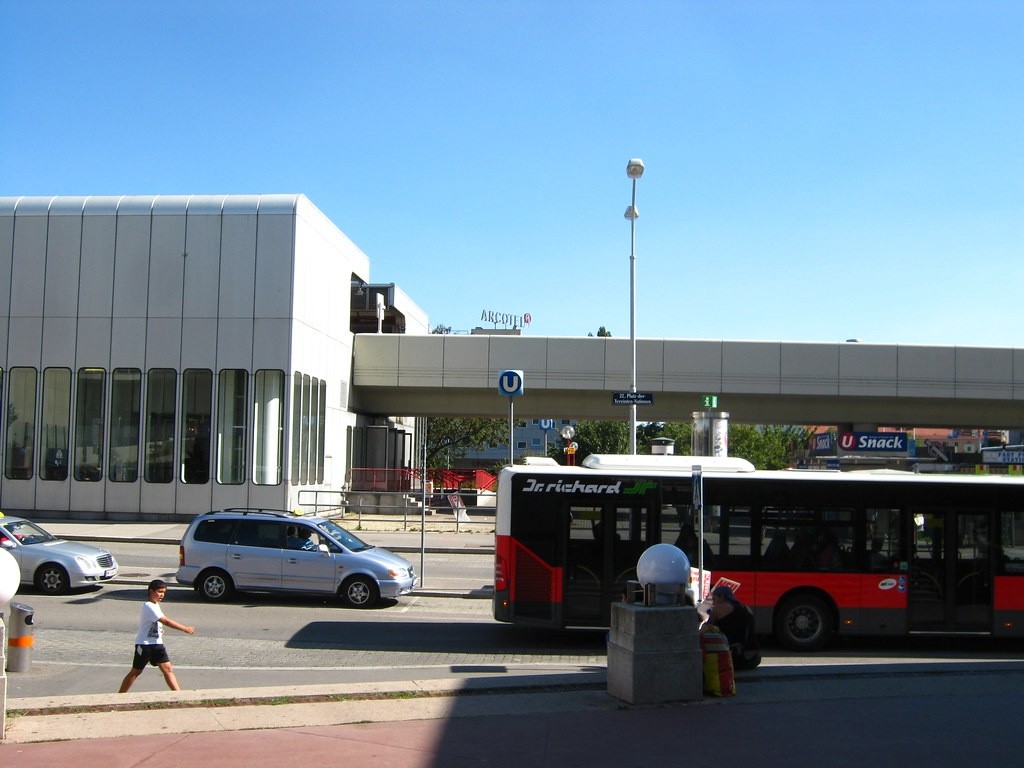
[592,521,603,544]
[287,526,297,547]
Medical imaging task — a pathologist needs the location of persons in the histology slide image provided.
[590,507,621,540]
[890,544,917,563]
[866,537,886,560]
[118,580,196,692]
[709,585,762,670]
[673,524,713,555]
[0,525,24,544]
[766,534,790,556]
[789,527,839,562]
[297,528,317,551]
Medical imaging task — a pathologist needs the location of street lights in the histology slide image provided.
[562,426,578,465]
[624,158,645,454]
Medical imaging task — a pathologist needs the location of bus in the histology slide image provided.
[492,453,1024,651]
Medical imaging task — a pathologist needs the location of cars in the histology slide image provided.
[0,515,118,595]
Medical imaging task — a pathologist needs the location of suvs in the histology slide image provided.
[174,507,418,608]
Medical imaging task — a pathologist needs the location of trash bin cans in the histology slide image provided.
[7,602,35,673]
